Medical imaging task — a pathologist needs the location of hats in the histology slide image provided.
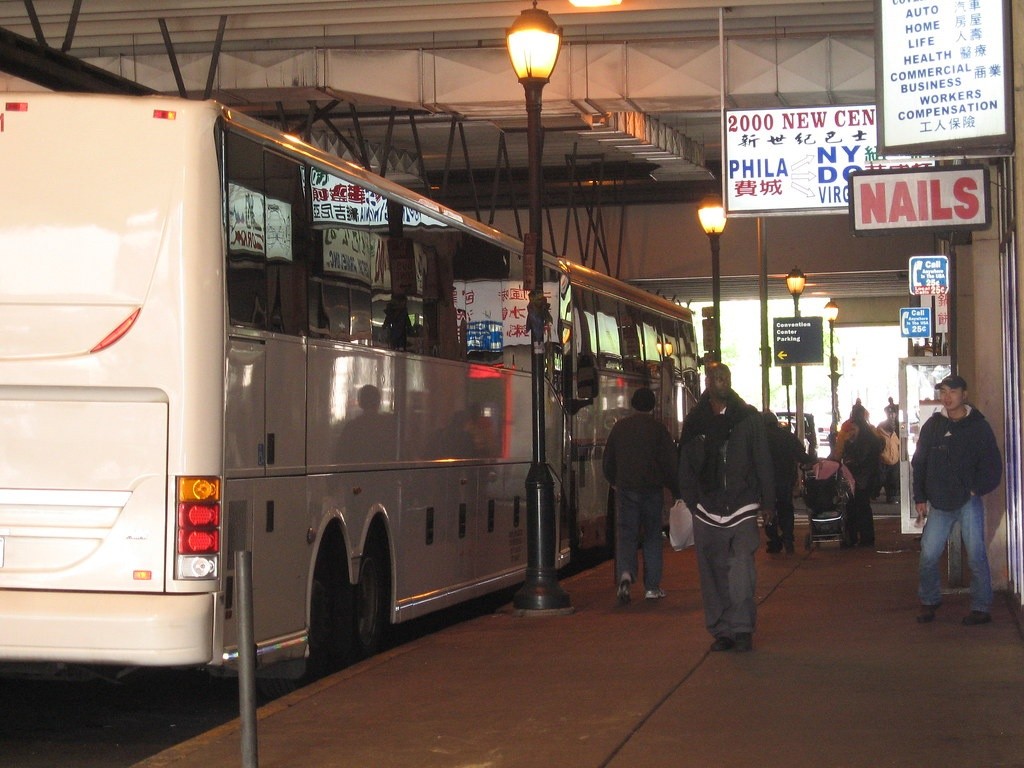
[935,375,968,390]
[629,388,656,412]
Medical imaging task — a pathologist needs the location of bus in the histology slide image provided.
[0,88,719,702]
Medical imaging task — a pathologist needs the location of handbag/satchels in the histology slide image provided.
[812,460,857,504]
[669,499,696,552]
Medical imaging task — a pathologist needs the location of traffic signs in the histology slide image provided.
[773,317,825,367]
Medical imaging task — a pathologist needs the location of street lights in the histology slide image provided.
[786,265,809,497]
[507,0,576,615]
[823,300,843,432]
[698,188,727,362]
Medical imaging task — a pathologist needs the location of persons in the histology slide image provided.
[912,375,1003,625]
[601,387,676,604]
[828,396,900,549]
[760,411,815,556]
[676,362,777,653]
[327,383,401,560]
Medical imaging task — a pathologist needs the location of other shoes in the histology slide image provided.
[765,541,783,554]
[963,611,992,626]
[917,606,936,624]
[785,541,794,554]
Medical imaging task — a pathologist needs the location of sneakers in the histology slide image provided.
[645,587,667,599]
[730,631,753,650]
[617,580,633,605]
[710,637,730,652]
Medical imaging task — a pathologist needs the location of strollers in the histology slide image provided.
[798,457,855,551]
